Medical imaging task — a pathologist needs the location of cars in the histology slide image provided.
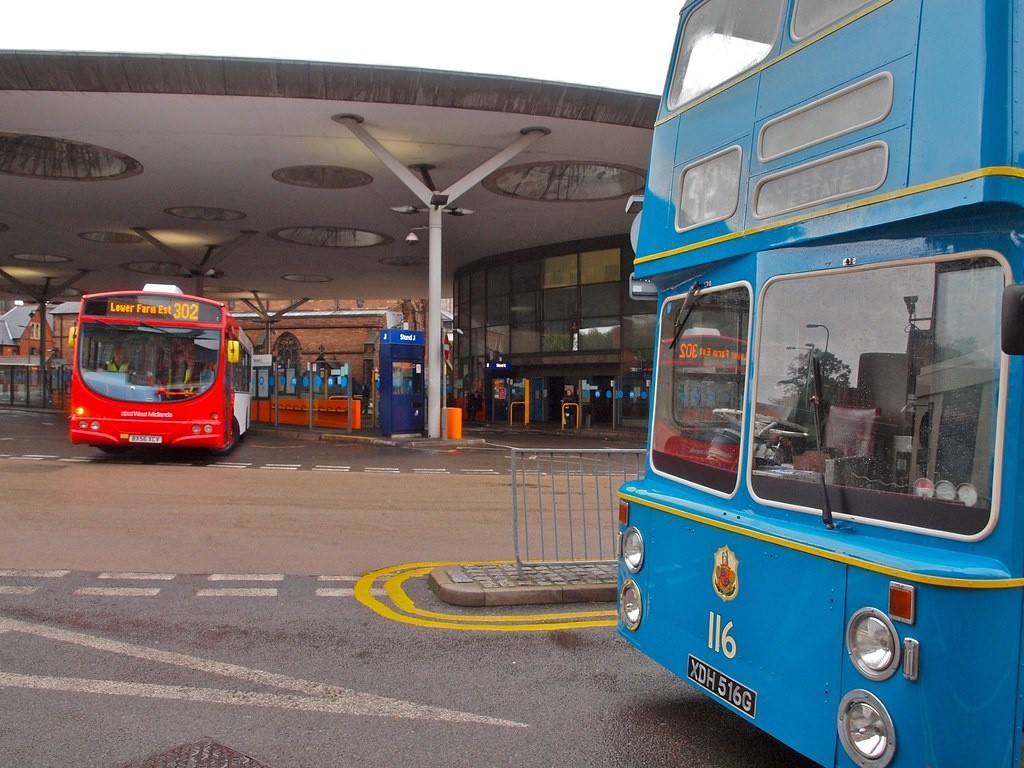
[656,422,757,473]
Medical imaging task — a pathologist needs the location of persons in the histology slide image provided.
[163,349,194,401]
[503,387,525,423]
[103,345,135,373]
[465,389,483,423]
[560,388,577,429]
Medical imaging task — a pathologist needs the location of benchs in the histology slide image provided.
[454,398,486,421]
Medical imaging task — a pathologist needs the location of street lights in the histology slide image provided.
[787,342,817,412]
[805,320,831,404]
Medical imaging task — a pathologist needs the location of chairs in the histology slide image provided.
[126,352,143,369]
[822,405,882,457]
[200,370,214,384]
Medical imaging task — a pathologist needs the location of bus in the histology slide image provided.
[67,284,257,454]
[615,0,1024,768]
